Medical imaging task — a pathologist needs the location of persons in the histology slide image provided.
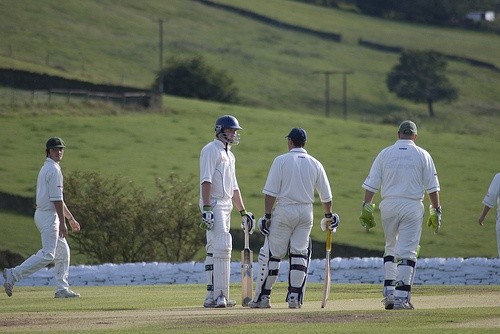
[248,128,339,308]
[360,120,441,310]
[0,137,80,299]
[478,172,500,257]
[200,115,256,308]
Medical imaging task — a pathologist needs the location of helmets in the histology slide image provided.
[215,115,243,145]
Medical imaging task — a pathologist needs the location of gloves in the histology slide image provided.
[427,205,442,235]
[239,210,256,235]
[257,213,271,236]
[200,205,215,231]
[320,213,341,232]
[359,200,377,232]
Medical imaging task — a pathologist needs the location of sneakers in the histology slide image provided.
[287,294,302,308]
[385,300,395,310]
[54,290,80,299]
[393,298,415,310]
[202,299,236,308]
[214,296,227,308]
[248,295,271,308]
[3,268,16,297]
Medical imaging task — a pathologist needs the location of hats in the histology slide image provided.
[284,127,307,147]
[45,137,65,148]
[397,119,418,135]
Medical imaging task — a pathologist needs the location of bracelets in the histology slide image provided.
[68,216,73,221]
